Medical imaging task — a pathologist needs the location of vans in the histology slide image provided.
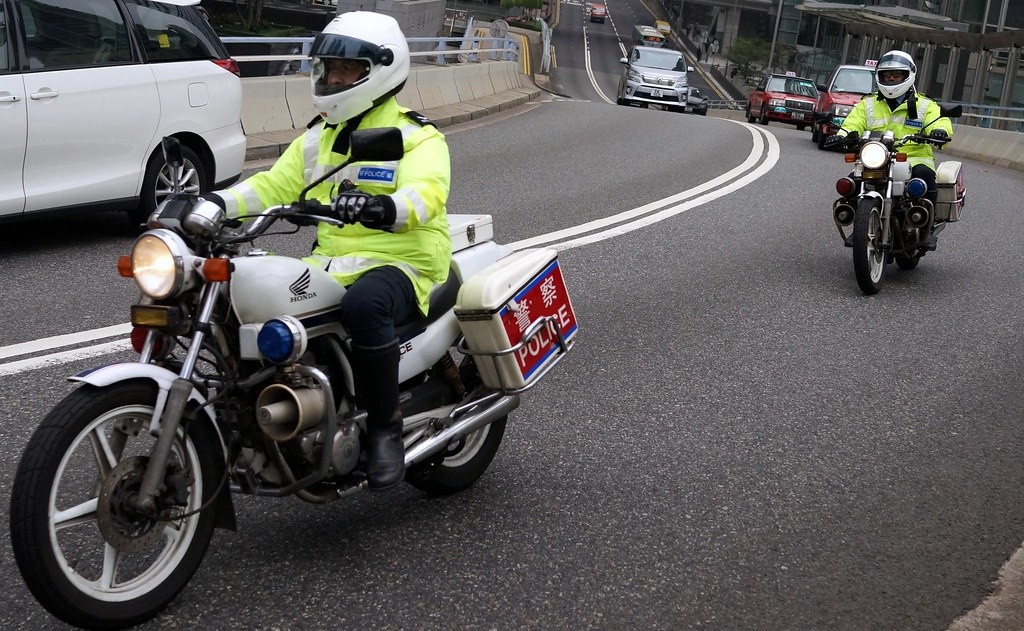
[655,21,672,41]
[617,45,694,112]
[590,4,607,24]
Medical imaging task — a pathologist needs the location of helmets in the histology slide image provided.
[308,10,411,125]
[874,50,916,98]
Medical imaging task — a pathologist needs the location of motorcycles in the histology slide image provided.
[810,102,964,296]
[7,126,579,631]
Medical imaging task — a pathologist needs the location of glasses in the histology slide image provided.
[881,71,905,78]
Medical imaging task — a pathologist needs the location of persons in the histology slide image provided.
[664,0,681,21]
[826,50,953,251]
[198,9,451,491]
[686,22,719,62]
[730,68,738,85]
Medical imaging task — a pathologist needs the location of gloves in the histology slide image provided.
[329,188,389,230]
[926,129,949,149]
[824,134,844,150]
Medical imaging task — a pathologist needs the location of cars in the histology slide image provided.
[685,86,709,115]
[746,70,816,131]
[811,61,885,151]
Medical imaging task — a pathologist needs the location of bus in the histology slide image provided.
[631,25,667,49]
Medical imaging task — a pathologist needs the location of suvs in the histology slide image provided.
[0,0,248,236]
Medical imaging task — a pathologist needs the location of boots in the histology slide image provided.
[843,231,853,248]
[919,190,936,252]
[350,336,406,491]
